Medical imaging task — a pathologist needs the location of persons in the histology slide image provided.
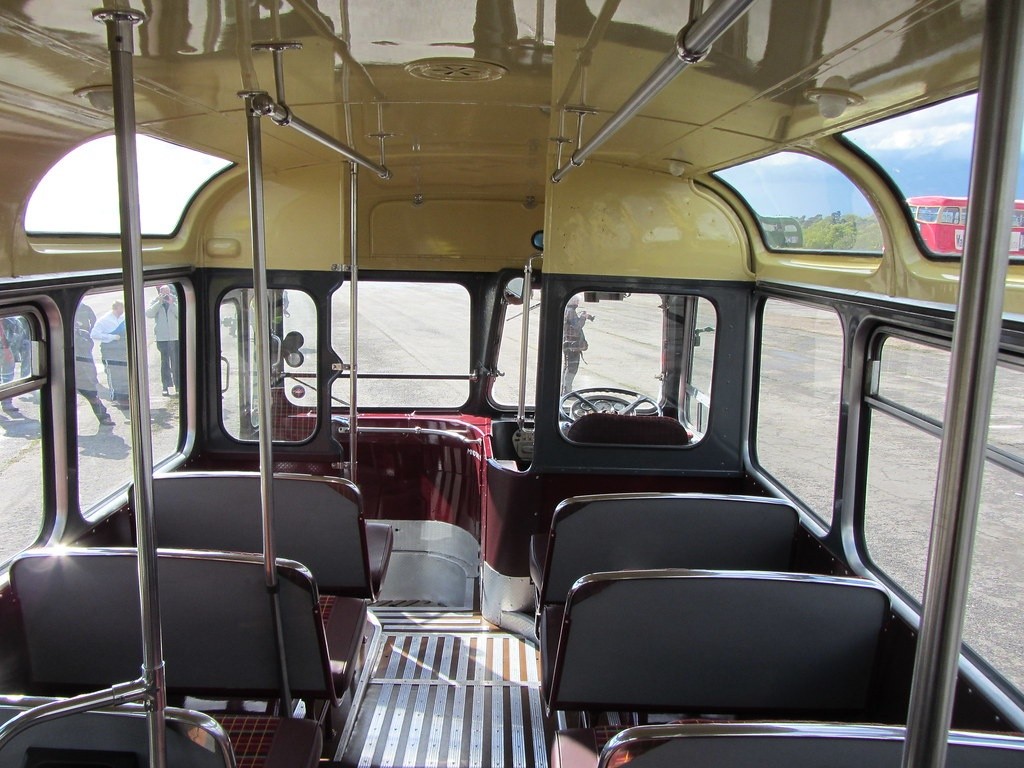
[561,295,588,400]
[0,282,179,426]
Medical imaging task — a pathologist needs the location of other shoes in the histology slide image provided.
[162,387,169,396]
[99,416,115,426]
[2,403,18,412]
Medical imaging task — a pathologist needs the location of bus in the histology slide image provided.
[906,195,1024,260]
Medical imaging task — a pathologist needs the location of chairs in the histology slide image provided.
[525,414,1023,768]
[0,471,396,768]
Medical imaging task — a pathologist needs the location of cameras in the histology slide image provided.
[584,310,595,321]
